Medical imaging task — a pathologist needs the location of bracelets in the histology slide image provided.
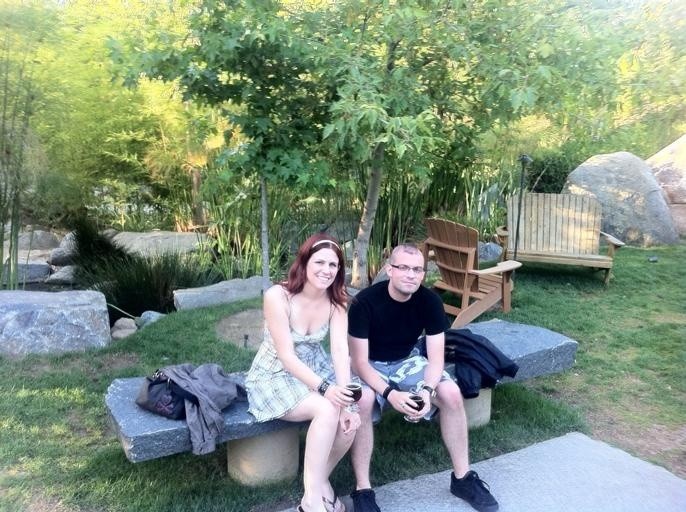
[382,386,396,399]
[318,377,331,396]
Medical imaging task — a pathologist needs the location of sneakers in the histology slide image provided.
[446,468,501,512]
[349,488,381,512]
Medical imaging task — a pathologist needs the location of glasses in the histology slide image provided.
[390,263,425,274]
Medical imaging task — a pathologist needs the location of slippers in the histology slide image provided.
[296,490,346,512]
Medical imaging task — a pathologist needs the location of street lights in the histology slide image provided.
[510,154,536,278]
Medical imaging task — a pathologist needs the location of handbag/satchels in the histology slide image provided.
[134,369,199,421]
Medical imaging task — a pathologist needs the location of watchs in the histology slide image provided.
[421,385,438,399]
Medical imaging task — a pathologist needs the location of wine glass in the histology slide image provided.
[344,374,363,413]
[403,387,424,424]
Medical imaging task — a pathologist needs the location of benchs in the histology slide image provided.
[102,317,580,492]
[494,190,626,289]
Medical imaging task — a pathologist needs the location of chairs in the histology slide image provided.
[418,216,523,331]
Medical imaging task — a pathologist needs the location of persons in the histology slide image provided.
[243,234,362,511]
[348,245,500,511]
